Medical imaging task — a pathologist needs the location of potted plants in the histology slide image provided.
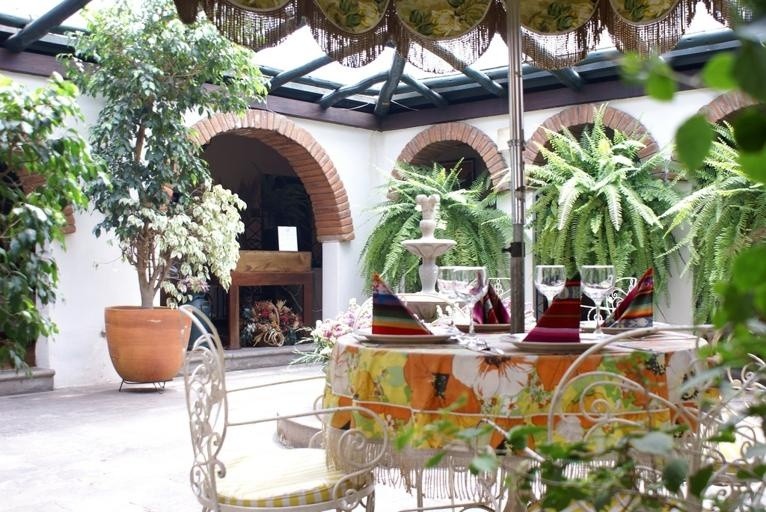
[54,0,268,383]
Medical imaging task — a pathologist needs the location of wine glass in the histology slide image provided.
[453,268,487,344]
[533,266,568,306]
[437,265,471,328]
[580,266,615,342]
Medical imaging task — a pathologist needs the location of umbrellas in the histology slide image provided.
[175,1,766,335]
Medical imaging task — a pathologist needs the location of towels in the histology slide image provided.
[370,269,433,336]
[474,271,511,325]
[600,266,653,328]
[521,270,580,342]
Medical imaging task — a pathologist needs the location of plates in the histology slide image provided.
[455,322,515,333]
[362,329,455,342]
[504,332,605,351]
[579,319,670,336]
